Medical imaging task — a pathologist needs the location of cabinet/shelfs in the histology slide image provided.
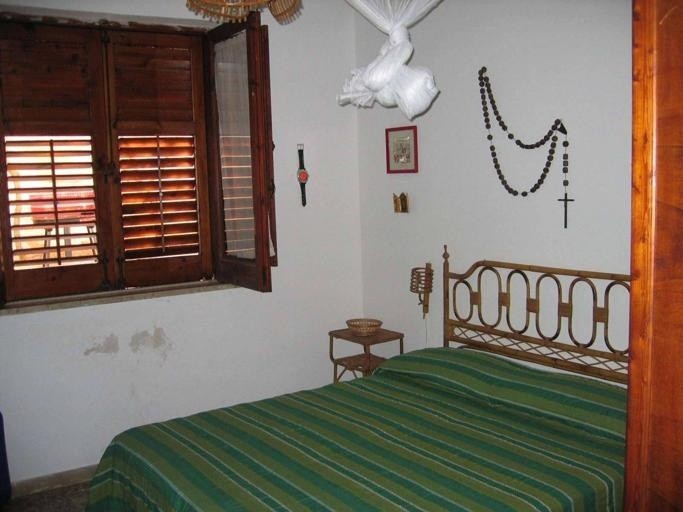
[327,328,403,385]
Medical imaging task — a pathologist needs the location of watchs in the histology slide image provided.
[296,144,308,206]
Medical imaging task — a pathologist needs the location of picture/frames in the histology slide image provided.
[384,126,418,173]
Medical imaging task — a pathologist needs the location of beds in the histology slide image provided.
[104,244,631,508]
